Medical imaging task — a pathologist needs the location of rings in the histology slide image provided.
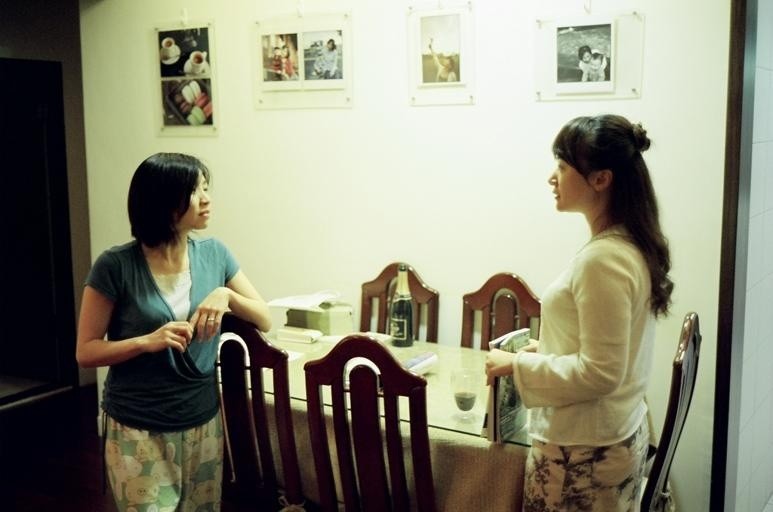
[206,318,214,320]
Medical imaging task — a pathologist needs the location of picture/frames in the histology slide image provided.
[535,11,643,98]
[252,11,354,109]
[154,20,220,136]
[405,0,476,106]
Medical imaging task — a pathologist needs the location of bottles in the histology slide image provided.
[390,264,413,346]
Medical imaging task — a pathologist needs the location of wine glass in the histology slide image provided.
[452,371,478,423]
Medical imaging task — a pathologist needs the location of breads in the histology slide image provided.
[173,81,212,125]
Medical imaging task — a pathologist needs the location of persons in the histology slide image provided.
[577,45,607,82]
[75,153,272,512]
[429,44,457,82]
[581,53,605,81]
[272,46,282,80]
[485,115,675,512]
[323,38,338,78]
[282,45,295,78]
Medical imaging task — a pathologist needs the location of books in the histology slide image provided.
[481,328,531,443]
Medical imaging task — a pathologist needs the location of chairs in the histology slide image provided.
[459,273,541,353]
[307,335,437,511]
[217,311,303,511]
[359,262,439,341]
[638,312,703,510]
